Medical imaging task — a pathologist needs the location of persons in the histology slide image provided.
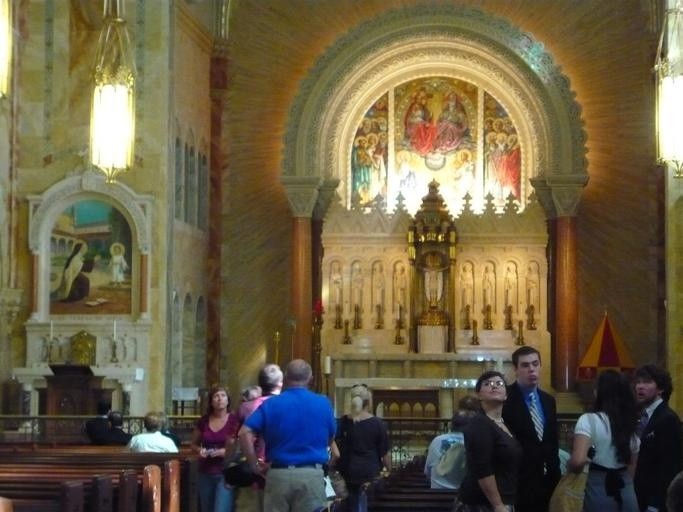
[107,411,135,447]
[47,239,101,304]
[424,346,682,512]
[83,418,121,448]
[124,414,177,452]
[157,412,181,445]
[415,258,450,310]
[190,359,391,512]
[345,84,521,203]
[106,243,131,287]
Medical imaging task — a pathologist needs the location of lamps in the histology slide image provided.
[648,0,683,183]
[84,1,140,186]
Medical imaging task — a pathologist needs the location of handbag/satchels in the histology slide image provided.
[225,463,258,487]
[549,444,596,512]
[438,443,469,481]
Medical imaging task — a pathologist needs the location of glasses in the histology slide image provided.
[482,380,505,386]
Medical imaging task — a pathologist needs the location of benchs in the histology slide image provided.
[313,444,466,512]
[0,416,204,512]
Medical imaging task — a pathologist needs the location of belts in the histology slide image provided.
[271,464,315,468]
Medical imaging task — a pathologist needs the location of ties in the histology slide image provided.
[529,393,544,442]
